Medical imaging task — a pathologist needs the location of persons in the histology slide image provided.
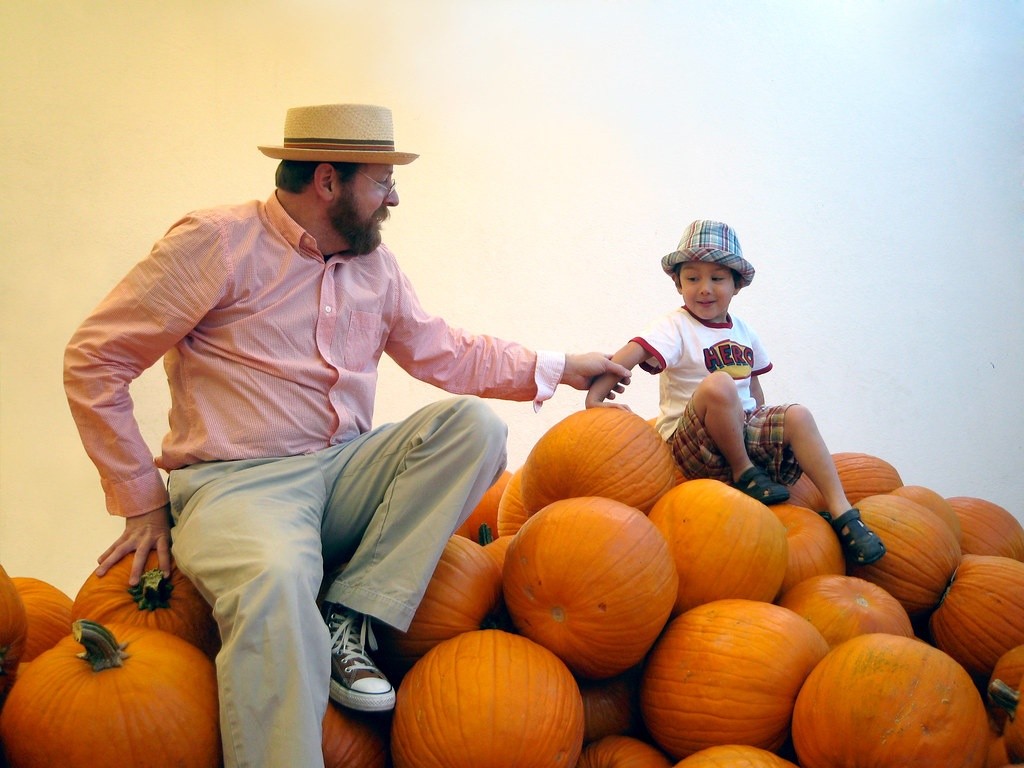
[63,104,632,768]
[585,219,886,565]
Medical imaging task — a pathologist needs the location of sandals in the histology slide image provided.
[728,464,791,504]
[826,506,887,566]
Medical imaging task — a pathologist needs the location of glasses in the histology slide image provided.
[358,169,397,196]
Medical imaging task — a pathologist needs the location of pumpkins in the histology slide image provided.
[0,406,1024,768]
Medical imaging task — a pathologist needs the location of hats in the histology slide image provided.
[257,102,420,165]
[661,220,754,287]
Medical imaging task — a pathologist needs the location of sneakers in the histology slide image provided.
[317,598,397,711]
